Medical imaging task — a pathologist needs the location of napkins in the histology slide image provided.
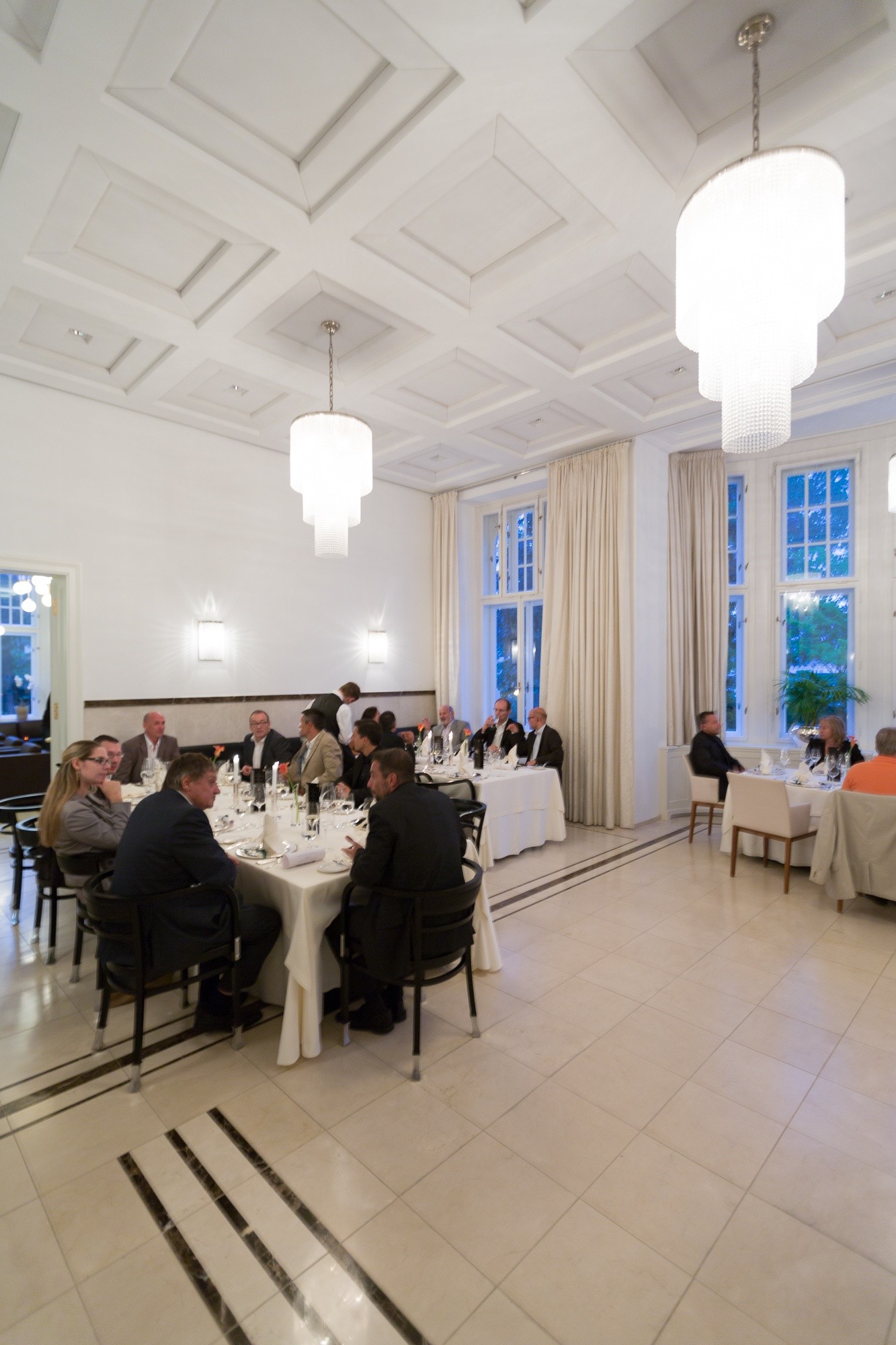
[262,812,289,853]
[758,748,824,785]
[414,743,519,777]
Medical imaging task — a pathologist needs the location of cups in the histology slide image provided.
[216,761,233,786]
[490,714,499,725]
[320,782,377,819]
[414,734,502,770]
[139,757,172,793]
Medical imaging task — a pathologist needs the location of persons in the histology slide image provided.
[690,712,745,800]
[379,710,415,762]
[806,716,864,766]
[506,707,564,781]
[94,734,123,774]
[111,711,180,784]
[842,726,896,795]
[239,710,291,782]
[288,709,342,795]
[334,748,476,1034]
[107,753,282,1031]
[334,718,391,807]
[360,707,381,724]
[37,740,131,905]
[471,699,525,758]
[299,682,360,746]
[422,705,474,754]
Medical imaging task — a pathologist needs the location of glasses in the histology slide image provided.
[492,708,507,713]
[108,752,124,759]
[527,717,535,721]
[252,720,268,726]
[87,756,110,766]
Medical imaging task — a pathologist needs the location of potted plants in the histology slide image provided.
[771,663,873,749]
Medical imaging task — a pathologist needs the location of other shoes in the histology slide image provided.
[334,1004,394,1035]
[195,1005,264,1032]
[388,999,407,1023]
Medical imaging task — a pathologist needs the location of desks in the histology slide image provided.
[413,758,568,870]
[719,766,844,867]
[117,780,503,1065]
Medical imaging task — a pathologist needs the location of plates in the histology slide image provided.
[316,860,353,873]
[784,780,828,788]
[211,817,298,859]
[447,772,481,779]
[749,767,785,776]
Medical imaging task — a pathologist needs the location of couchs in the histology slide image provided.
[0,732,50,822]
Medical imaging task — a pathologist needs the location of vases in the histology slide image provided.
[15,705,29,722]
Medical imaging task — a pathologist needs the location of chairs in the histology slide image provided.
[0,792,244,1094]
[683,751,896,915]
[341,771,487,1082]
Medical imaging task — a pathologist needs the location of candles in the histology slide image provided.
[427,730,432,751]
[448,730,453,756]
[233,754,279,793]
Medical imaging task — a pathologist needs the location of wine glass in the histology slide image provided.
[306,802,319,839]
[780,748,848,792]
[241,783,265,822]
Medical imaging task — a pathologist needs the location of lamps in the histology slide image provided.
[368,630,389,664]
[674,13,896,513]
[290,320,373,558]
[197,621,224,662]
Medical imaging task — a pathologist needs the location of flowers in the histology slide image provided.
[848,736,858,753]
[13,673,36,707]
[212,745,224,764]
[417,722,425,744]
[279,761,299,825]
[464,727,471,756]
